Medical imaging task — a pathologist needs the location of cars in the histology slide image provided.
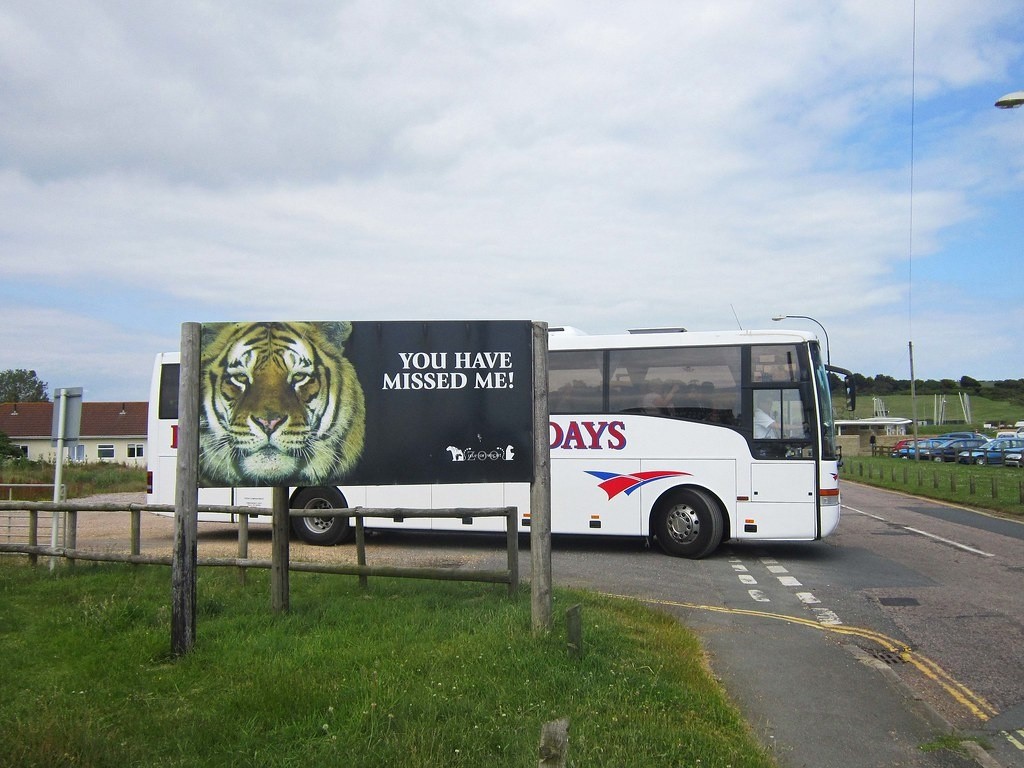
[890,427,1024,467]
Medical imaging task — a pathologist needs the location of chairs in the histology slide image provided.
[548,380,713,422]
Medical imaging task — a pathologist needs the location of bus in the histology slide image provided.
[143,324,855,560]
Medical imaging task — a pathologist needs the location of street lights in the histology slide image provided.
[771,315,831,392]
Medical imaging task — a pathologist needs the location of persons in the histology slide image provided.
[753,392,810,439]
[868,431,877,454]
[549,377,730,422]
